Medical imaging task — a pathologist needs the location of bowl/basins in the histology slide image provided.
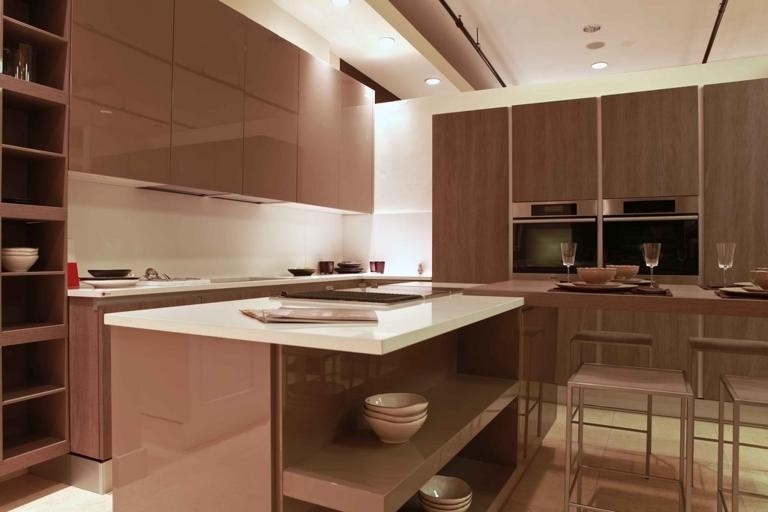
[1,247,38,273]
[606,265,640,281]
[361,393,429,444]
[575,268,617,284]
[419,475,473,512]
[750,271,768,290]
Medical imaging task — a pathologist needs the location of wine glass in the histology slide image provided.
[716,243,736,287]
[561,242,577,284]
[643,243,661,289]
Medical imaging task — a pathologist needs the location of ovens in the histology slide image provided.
[511,196,700,278]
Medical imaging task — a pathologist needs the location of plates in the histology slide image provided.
[335,268,363,273]
[719,286,768,297]
[80,278,140,288]
[288,269,315,276]
[338,263,361,268]
[87,270,132,278]
[556,283,639,292]
[628,279,656,285]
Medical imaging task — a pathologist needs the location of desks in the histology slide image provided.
[466,280,767,383]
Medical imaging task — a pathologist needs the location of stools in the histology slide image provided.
[565,363,696,512]
[717,372,768,511]
[573,330,654,367]
[687,337,767,385]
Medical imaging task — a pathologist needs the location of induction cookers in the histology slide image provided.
[281,289,422,303]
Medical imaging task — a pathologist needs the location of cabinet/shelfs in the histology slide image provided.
[103,283,526,511]
[1,0,73,511]
